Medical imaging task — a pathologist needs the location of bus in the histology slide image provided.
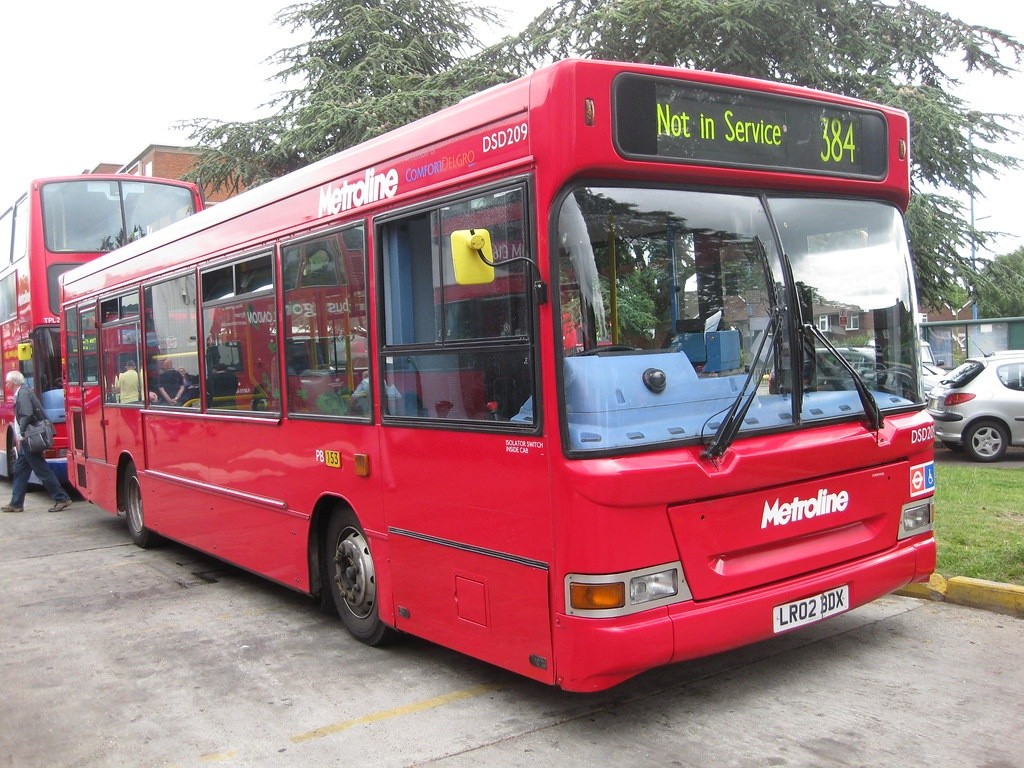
[0,175,205,487]
[53,60,935,696]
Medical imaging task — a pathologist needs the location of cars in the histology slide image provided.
[804,336,952,405]
[926,351,1024,461]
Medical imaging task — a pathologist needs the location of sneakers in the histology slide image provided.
[0,503,24,513]
[48,499,72,513]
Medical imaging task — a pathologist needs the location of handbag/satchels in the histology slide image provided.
[19,418,54,455]
[37,419,57,437]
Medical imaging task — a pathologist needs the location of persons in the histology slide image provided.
[98,293,410,421]
[0,369,73,513]
